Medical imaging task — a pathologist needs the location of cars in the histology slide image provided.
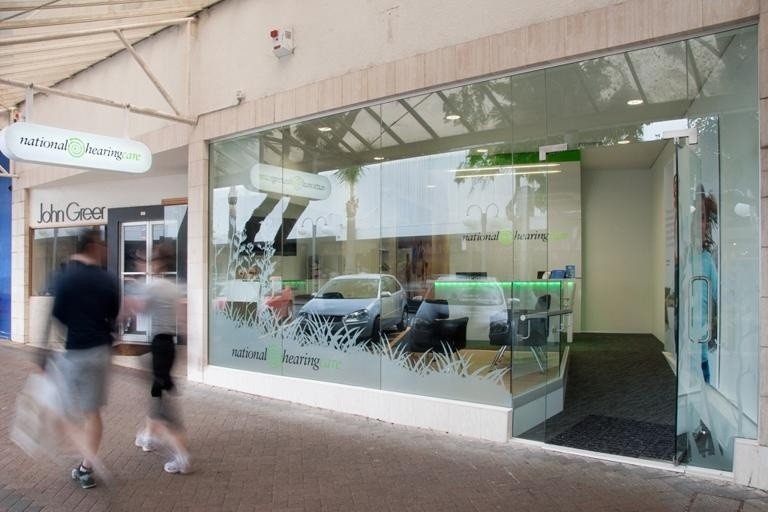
[121,276,188,344]
[297,273,410,346]
[410,275,510,354]
[214,275,295,327]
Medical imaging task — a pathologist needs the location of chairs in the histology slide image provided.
[395,299,469,371]
[488,294,552,375]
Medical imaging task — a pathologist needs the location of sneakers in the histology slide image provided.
[72,468,96,489]
[134,435,153,452]
[163,458,192,474]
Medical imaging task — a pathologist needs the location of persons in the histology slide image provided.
[404,239,430,287]
[677,183,723,387]
[121,243,198,476]
[31,224,122,491]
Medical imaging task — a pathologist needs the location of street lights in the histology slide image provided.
[296,214,332,294]
[37,227,74,294]
[687,172,752,220]
[462,202,503,274]
